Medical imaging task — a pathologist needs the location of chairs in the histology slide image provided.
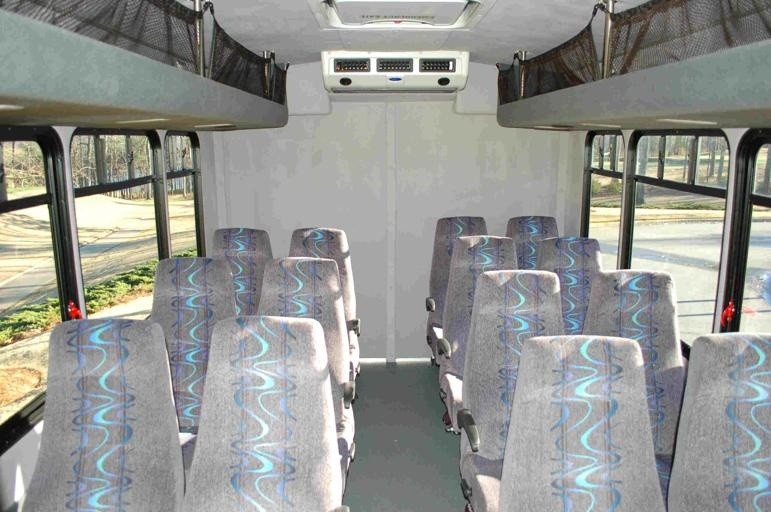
[425,215,771,512]
[25,227,361,512]
